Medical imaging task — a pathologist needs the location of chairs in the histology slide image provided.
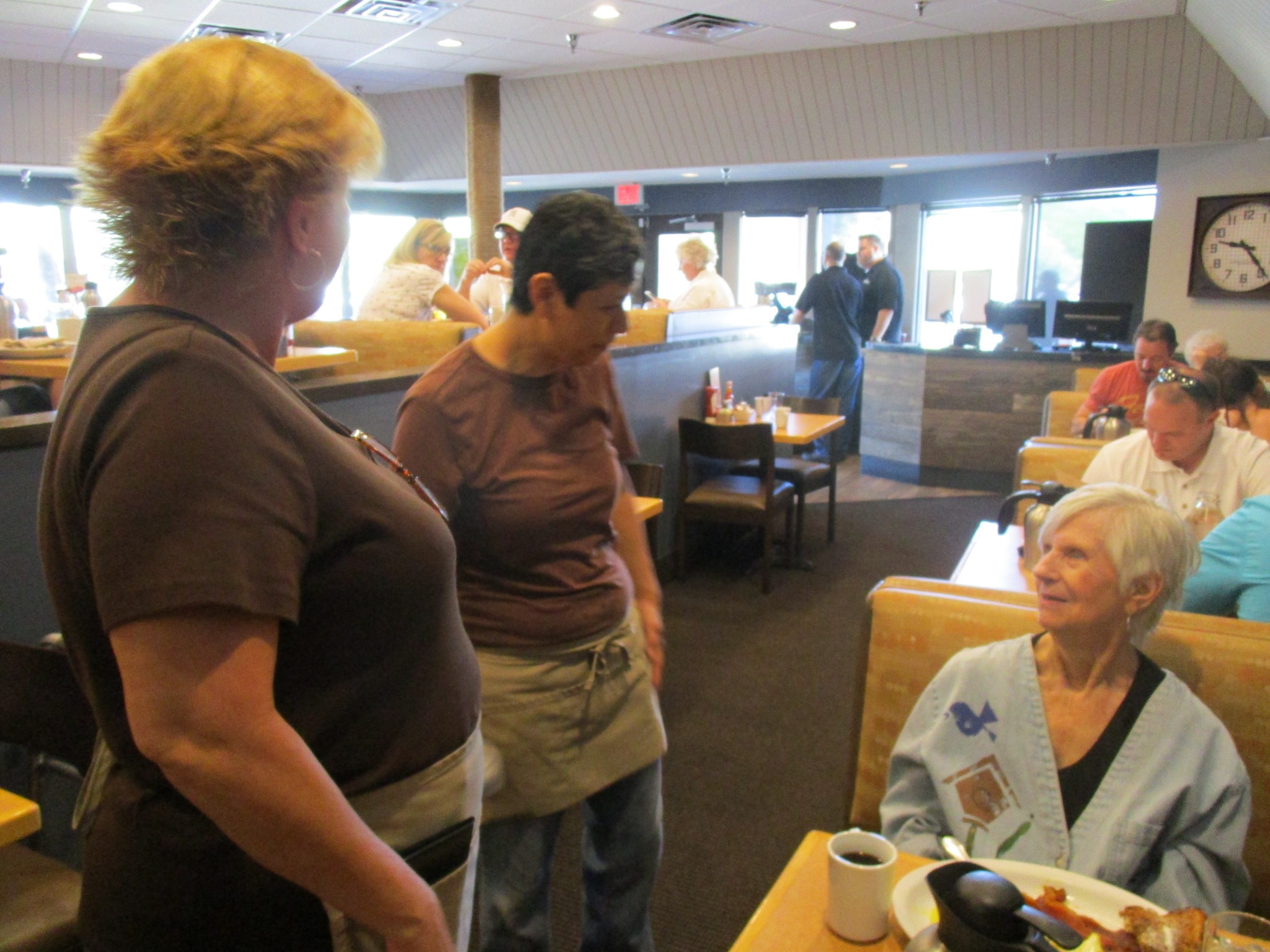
[672,416,800,593]
[733,390,841,558]
[0,640,97,952]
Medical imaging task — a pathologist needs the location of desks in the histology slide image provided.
[0,345,357,379]
[703,407,847,569]
[949,519,1038,596]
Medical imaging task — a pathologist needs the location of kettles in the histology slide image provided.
[0,281,20,341]
[996,477,1076,572]
[81,280,102,308]
[1081,404,1132,442]
[901,860,1087,952]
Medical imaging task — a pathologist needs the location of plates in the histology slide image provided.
[1,346,71,358]
[892,857,1171,952]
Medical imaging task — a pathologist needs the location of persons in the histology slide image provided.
[38,35,481,952]
[357,206,1270,627]
[391,189,666,952]
[881,482,1255,934]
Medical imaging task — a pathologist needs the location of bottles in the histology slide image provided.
[723,381,734,409]
[1185,490,1226,541]
[704,386,715,424]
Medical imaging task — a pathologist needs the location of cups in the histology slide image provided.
[55,318,85,344]
[825,827,899,945]
[1199,909,1270,952]
[488,291,507,327]
[276,324,294,357]
[776,406,792,428]
[754,396,772,417]
[769,392,785,414]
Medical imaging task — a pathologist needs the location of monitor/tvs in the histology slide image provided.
[1052,299,1132,353]
[1003,300,1045,351]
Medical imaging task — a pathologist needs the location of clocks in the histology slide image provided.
[1187,192,1270,301]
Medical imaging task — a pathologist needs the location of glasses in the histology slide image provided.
[420,244,450,256]
[494,230,522,239]
[1157,368,1214,408]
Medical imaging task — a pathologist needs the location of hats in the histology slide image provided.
[493,207,533,233]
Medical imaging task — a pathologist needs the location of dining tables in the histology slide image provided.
[729,829,1268,952]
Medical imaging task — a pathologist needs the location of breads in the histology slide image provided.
[1133,909,1209,952]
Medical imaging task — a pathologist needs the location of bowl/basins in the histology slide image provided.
[732,409,753,421]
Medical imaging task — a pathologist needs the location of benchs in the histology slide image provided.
[852,367,1269,939]
[289,310,668,383]
